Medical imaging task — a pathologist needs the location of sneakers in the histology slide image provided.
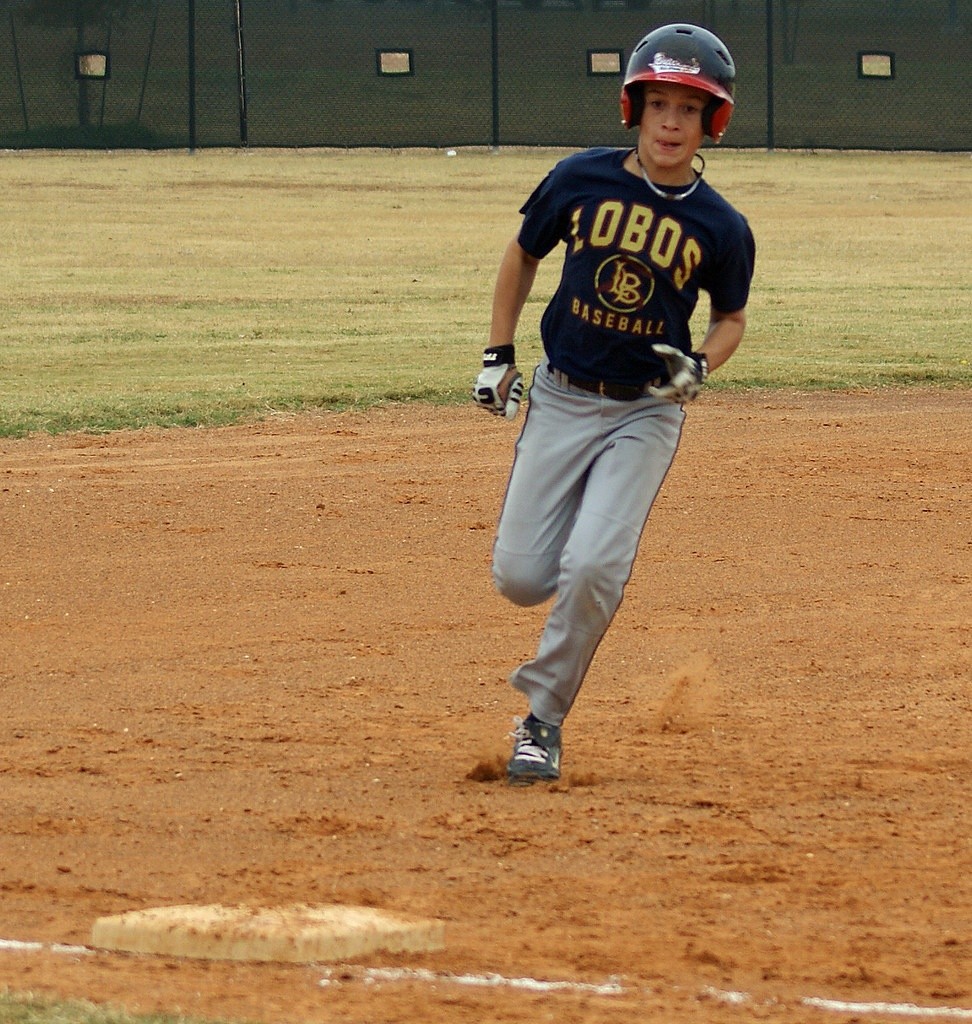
[508,712,560,778]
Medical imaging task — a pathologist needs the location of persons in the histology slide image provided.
[472,23,756,780]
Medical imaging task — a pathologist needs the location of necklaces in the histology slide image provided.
[636,148,705,199]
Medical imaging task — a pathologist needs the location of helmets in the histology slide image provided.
[621,24,736,143]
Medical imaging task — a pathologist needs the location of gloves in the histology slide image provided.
[472,344,523,421]
[646,345,708,397]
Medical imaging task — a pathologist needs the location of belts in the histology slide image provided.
[548,362,671,401]
